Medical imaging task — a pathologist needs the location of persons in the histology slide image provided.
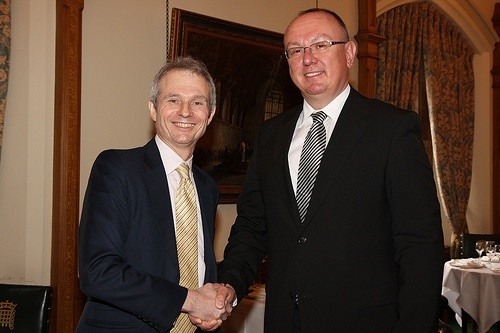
[75,56,235,333]
[187,8,445,333]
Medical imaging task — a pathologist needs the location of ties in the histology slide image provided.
[296,111,328,304]
[170,162,199,333]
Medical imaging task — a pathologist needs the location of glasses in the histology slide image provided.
[285,40,348,59]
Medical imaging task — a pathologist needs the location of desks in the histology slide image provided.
[220,283,266,333]
[440,255,500,333]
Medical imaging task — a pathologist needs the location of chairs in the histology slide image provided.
[0,284,54,333]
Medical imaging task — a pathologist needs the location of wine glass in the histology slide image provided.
[475,241,484,261]
[485,241,500,264]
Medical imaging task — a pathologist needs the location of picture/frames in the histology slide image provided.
[168,8,304,201]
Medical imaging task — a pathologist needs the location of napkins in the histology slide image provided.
[448,256,500,273]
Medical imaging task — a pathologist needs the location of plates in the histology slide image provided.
[449,259,485,267]
